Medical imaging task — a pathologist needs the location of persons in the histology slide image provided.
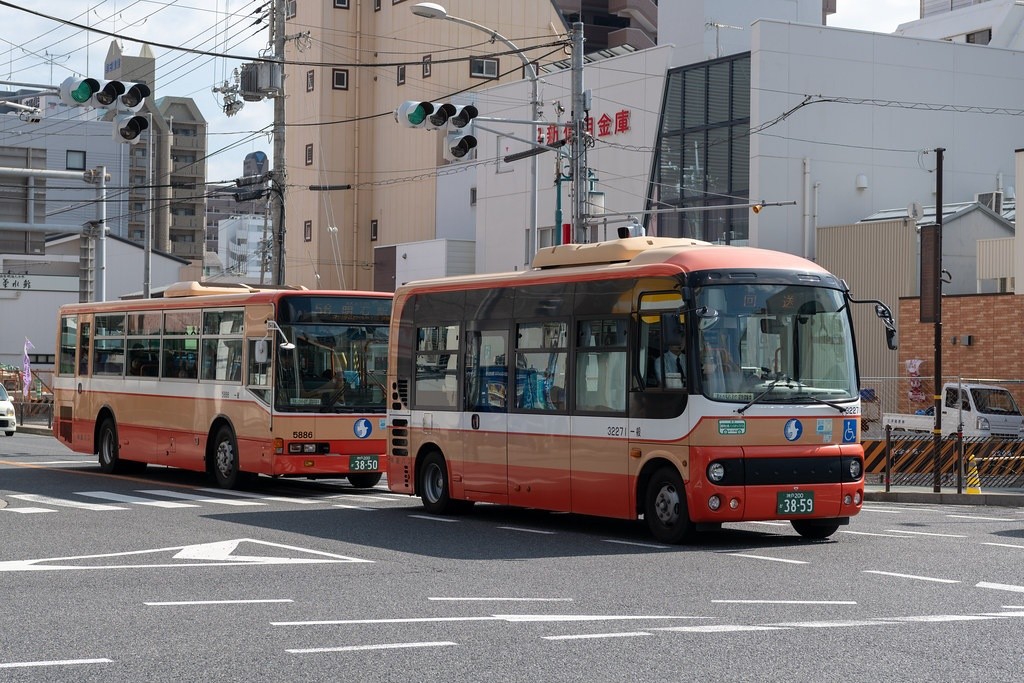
[275,351,306,394]
[655,330,718,391]
[73,338,225,381]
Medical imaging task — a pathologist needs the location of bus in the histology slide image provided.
[384,233,899,543]
[51,280,397,489]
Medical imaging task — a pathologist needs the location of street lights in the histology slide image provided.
[411,2,540,275]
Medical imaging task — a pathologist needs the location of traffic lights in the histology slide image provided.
[397,100,477,163]
[60,75,152,146]
[919,223,940,324]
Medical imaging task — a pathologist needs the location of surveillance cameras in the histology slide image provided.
[617,224,645,239]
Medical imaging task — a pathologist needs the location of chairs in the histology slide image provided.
[63,344,218,380]
[416,352,616,414]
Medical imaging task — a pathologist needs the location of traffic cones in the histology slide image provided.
[966,454,981,493]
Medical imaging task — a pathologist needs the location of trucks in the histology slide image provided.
[882,381,1024,442]
[859,387,881,431]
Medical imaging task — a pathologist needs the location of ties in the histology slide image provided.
[676,357,684,383]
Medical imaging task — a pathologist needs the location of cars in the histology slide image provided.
[0,382,16,436]
